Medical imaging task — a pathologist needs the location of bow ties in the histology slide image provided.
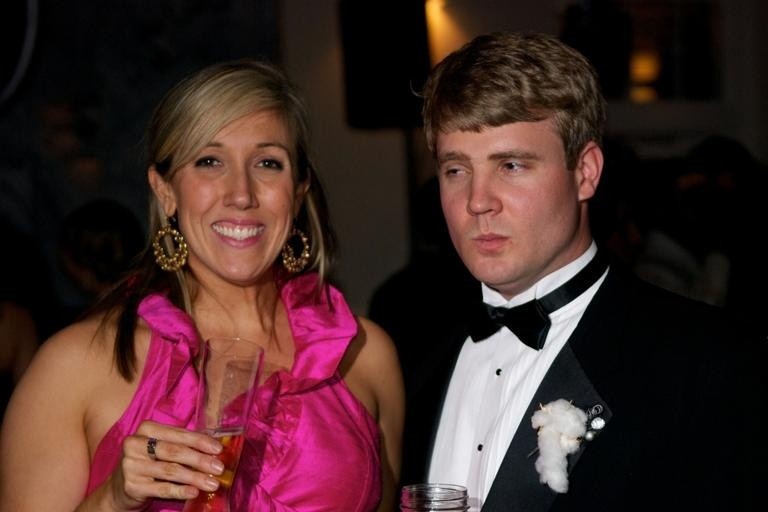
[457,293,551,351]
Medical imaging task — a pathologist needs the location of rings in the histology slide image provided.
[147,436,156,459]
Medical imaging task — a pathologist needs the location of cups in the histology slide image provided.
[400,482,472,511]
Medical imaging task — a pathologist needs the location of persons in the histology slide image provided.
[371,30,767,509]
[3,99,154,388]
[0,61,406,512]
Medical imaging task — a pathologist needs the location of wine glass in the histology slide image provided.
[180,336,265,510]
